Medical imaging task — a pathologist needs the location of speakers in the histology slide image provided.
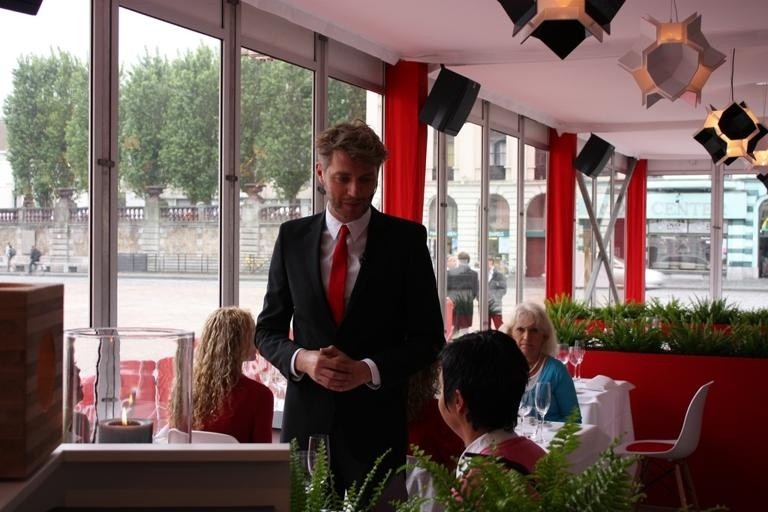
[420,68,481,136]
[574,134,615,178]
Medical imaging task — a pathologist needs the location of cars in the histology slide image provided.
[653,254,711,270]
[541,255,664,290]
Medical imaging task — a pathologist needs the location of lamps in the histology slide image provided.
[615,1,729,111]
[746,114,768,191]
[492,1,629,62]
[690,44,762,169]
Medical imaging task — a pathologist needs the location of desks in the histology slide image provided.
[561,366,642,493]
[513,414,608,481]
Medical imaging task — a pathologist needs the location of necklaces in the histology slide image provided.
[528,352,543,376]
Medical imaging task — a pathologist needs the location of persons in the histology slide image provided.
[433,327,556,512]
[28,246,42,273]
[6,243,18,274]
[250,117,447,512]
[504,305,584,426]
[477,256,509,331]
[167,305,274,444]
[447,251,479,340]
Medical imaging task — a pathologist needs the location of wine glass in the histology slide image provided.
[569,339,585,382]
[536,380,551,443]
[517,382,532,436]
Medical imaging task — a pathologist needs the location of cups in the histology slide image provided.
[555,344,569,364]
[297,448,316,493]
[66,325,196,444]
[309,434,332,481]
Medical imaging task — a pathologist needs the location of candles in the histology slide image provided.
[94,408,154,443]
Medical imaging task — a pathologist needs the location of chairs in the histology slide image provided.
[611,378,717,510]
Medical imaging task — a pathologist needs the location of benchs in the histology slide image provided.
[0,261,88,275]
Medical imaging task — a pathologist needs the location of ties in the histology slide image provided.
[326,222,352,324]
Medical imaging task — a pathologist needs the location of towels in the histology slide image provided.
[583,370,613,394]
